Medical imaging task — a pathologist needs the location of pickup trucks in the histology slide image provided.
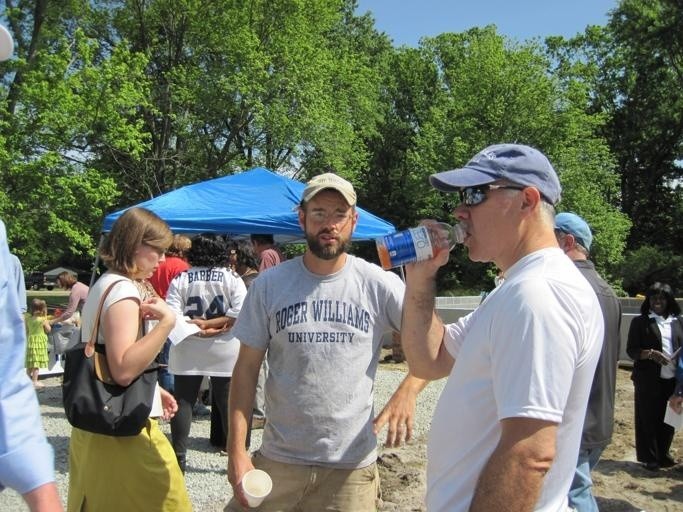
[26,272,56,290]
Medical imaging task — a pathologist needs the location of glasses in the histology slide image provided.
[141,240,166,256]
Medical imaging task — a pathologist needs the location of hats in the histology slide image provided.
[301,173,356,208]
[554,213,594,252]
[430,142,562,206]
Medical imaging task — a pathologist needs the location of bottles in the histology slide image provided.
[375,221,470,272]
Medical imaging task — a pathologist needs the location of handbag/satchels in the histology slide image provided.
[62,280,159,438]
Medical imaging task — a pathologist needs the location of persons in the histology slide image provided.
[223,173,433,512]
[49,271,90,325]
[22,298,52,393]
[663,355,682,430]
[626,281,683,472]
[164,232,249,474]
[547,212,622,511]
[62,206,195,512]
[226,238,260,287]
[375,143,604,512]
[250,234,283,272]
[1,217,67,511]
[147,234,211,415]
